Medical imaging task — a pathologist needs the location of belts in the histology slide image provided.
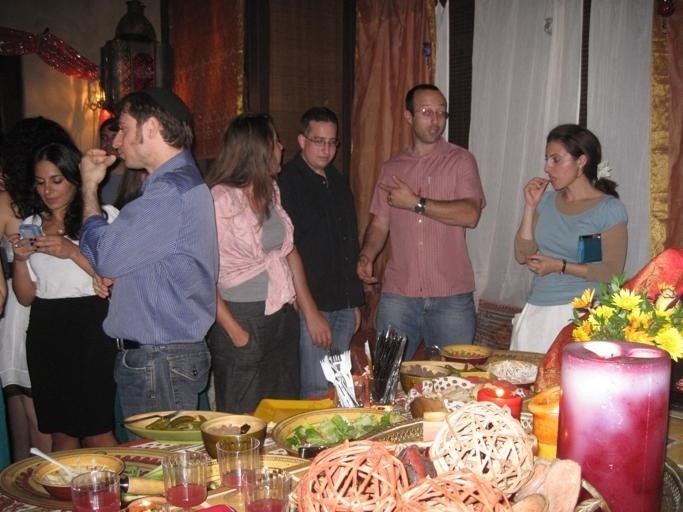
[113,339,140,352]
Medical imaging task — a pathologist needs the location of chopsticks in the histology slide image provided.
[364,331,406,400]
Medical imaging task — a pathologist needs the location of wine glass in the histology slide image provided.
[160,451,208,510]
[215,433,261,507]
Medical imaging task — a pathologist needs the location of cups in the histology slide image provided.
[241,467,291,512]
[333,381,365,407]
[69,471,121,512]
[368,374,399,408]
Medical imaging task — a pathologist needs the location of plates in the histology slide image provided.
[486,359,537,385]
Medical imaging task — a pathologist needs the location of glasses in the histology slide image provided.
[414,108,450,119]
[303,134,341,147]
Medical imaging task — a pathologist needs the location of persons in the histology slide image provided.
[508,123,627,355]
[278,106,365,402]
[356,83,486,362]
[203,111,302,415]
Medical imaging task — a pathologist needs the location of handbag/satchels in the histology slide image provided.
[576,234,602,264]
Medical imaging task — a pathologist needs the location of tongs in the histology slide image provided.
[299,417,424,458]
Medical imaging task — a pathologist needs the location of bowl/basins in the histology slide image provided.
[399,361,450,398]
[160,455,308,496]
[438,344,494,365]
[200,415,267,459]
[29,454,124,499]
[123,408,232,445]
[273,407,397,456]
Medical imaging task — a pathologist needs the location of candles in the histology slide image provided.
[476,388,522,421]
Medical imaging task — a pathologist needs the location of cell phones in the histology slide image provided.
[19,224,42,246]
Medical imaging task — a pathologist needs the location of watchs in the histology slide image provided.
[415,198,425,215]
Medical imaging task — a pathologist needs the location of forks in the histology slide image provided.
[318,350,357,407]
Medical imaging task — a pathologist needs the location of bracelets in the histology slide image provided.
[560,259,566,274]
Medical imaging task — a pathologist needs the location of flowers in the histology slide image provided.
[565,278,682,363]
